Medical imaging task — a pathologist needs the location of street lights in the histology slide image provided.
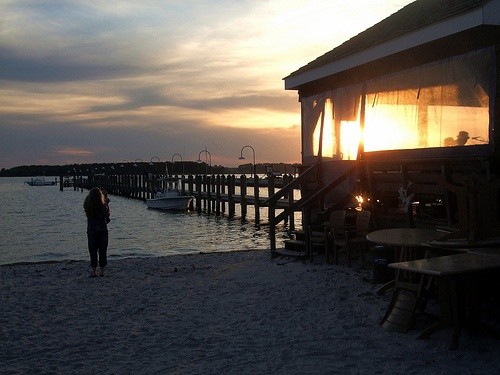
[66,143,255,169]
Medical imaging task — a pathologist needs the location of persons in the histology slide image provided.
[82,188,111,277]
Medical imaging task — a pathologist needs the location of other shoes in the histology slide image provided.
[89,274,105,277]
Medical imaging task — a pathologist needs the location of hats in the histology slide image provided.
[457,131,470,139]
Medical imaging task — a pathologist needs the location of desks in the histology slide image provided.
[365,227,450,294]
[388,250,498,354]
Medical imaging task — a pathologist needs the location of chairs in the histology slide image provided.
[307,209,345,264]
[332,210,371,266]
[378,245,442,334]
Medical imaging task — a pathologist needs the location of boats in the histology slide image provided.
[146,177,194,211]
[25,177,56,187]
